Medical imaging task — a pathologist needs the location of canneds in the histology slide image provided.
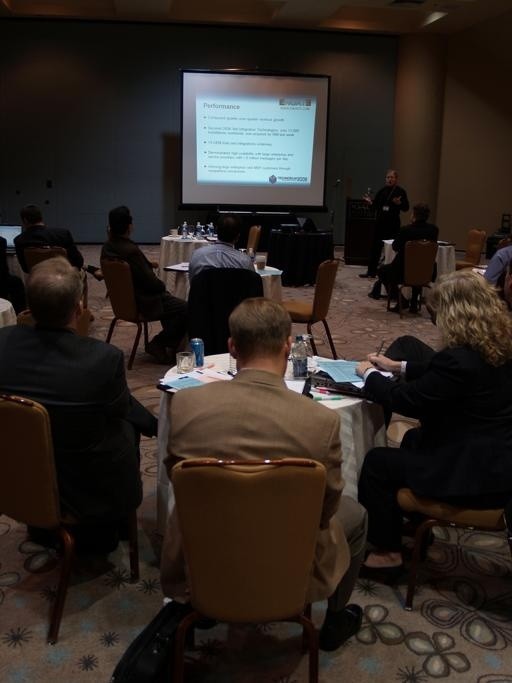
[190,338,204,367]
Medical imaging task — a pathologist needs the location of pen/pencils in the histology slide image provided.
[315,396,345,401]
[228,371,235,377]
[372,339,385,365]
[311,387,330,395]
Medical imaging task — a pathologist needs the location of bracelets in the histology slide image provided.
[361,366,374,375]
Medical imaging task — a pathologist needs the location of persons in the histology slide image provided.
[159,296,363,652]
[355,269,511,568]
[13,202,105,322]
[0,254,158,557]
[381,257,512,430]
[100,206,190,365]
[484,230,511,283]
[358,169,409,277]
[1,234,19,309]
[146,214,261,354]
[382,204,438,312]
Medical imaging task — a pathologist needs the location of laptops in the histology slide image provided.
[0,223,24,255]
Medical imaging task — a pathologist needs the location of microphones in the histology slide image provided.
[384,176,391,184]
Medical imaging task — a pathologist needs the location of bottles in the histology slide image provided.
[182,221,187,238]
[292,335,308,379]
[196,221,201,238]
[248,247,259,264]
[229,354,236,375]
[302,333,315,366]
[208,221,214,236]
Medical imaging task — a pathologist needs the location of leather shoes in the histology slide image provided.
[145,336,172,364]
[55,538,115,577]
[319,603,364,654]
[94,268,103,281]
[359,272,419,314]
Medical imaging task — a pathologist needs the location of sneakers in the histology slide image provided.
[402,518,435,548]
[360,549,408,584]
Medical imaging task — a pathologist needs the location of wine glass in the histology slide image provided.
[206,223,210,233]
[199,225,206,236]
[186,224,195,240]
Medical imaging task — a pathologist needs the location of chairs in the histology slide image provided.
[2,394,141,646]
[395,489,510,612]
[282,259,340,359]
[456,229,486,269]
[99,256,164,370]
[246,226,262,253]
[161,458,327,682]
[17,245,89,316]
[387,238,439,323]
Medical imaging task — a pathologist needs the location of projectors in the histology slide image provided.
[280,224,300,233]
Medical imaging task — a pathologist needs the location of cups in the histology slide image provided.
[175,350,193,375]
[256,255,266,270]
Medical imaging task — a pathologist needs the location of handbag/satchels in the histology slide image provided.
[110,601,194,681]
